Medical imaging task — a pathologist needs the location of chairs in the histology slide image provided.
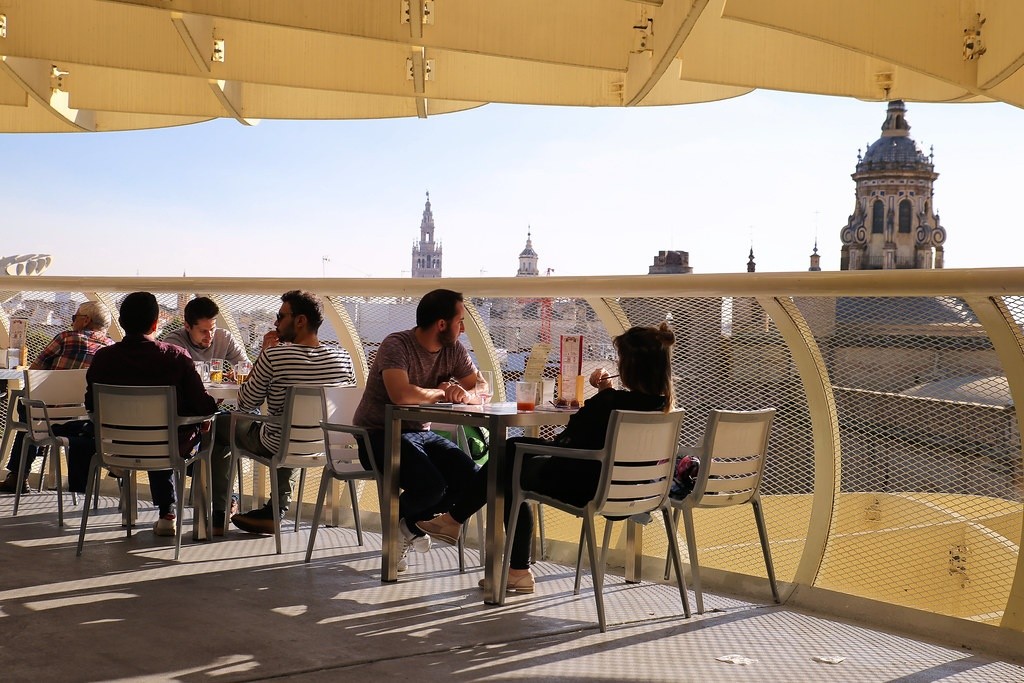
[0,368,781,632]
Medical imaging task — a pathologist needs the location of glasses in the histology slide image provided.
[72,314,92,323]
[276,311,296,323]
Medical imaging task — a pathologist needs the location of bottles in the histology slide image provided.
[542,377,555,405]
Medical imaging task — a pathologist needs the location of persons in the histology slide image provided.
[353,289,493,571]
[416,322,675,593]
[0,290,358,538]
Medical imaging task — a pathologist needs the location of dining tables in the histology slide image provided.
[381,401,585,605]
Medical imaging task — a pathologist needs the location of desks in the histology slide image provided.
[0,364,31,485]
[192,383,265,540]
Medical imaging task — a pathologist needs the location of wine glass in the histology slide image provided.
[475,371,494,407]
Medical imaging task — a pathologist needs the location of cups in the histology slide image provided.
[515,381,537,413]
[193,360,209,385]
[210,358,223,384]
[233,360,251,386]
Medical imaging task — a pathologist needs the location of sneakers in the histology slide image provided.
[399,518,431,571]
[228,499,285,535]
[479,567,535,593]
[153,513,175,536]
[416,515,463,546]
[0,472,31,493]
